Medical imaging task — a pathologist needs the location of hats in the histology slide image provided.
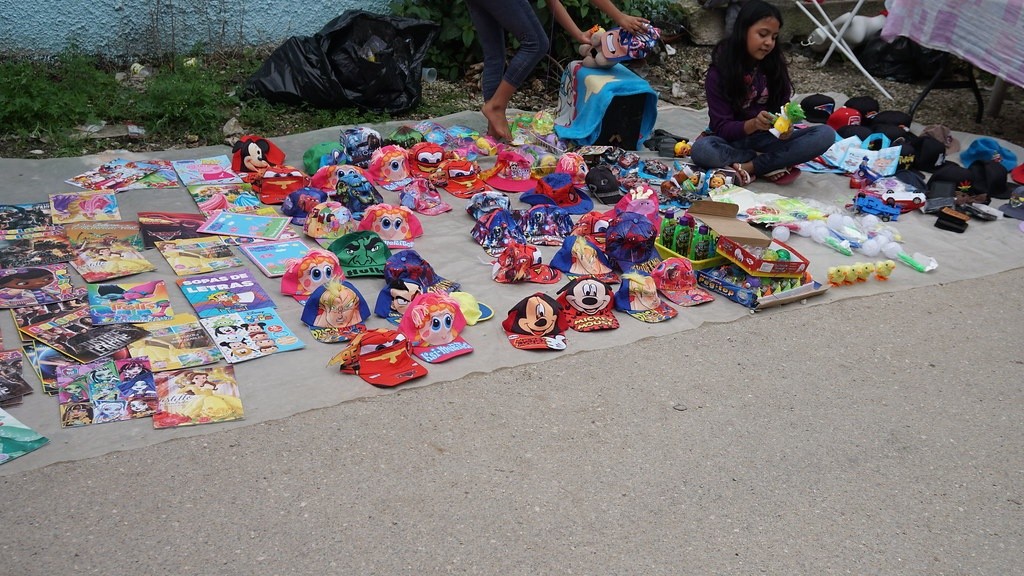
[233,122,714,390]
[601,20,670,65]
[799,86,1024,221]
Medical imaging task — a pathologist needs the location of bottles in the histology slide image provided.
[657,206,724,262]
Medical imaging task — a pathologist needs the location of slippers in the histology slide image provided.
[724,161,756,186]
[759,164,802,185]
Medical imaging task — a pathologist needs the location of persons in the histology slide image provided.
[690,0,836,188]
[464,0,650,142]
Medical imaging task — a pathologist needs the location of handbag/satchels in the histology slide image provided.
[237,9,447,126]
[855,31,974,88]
